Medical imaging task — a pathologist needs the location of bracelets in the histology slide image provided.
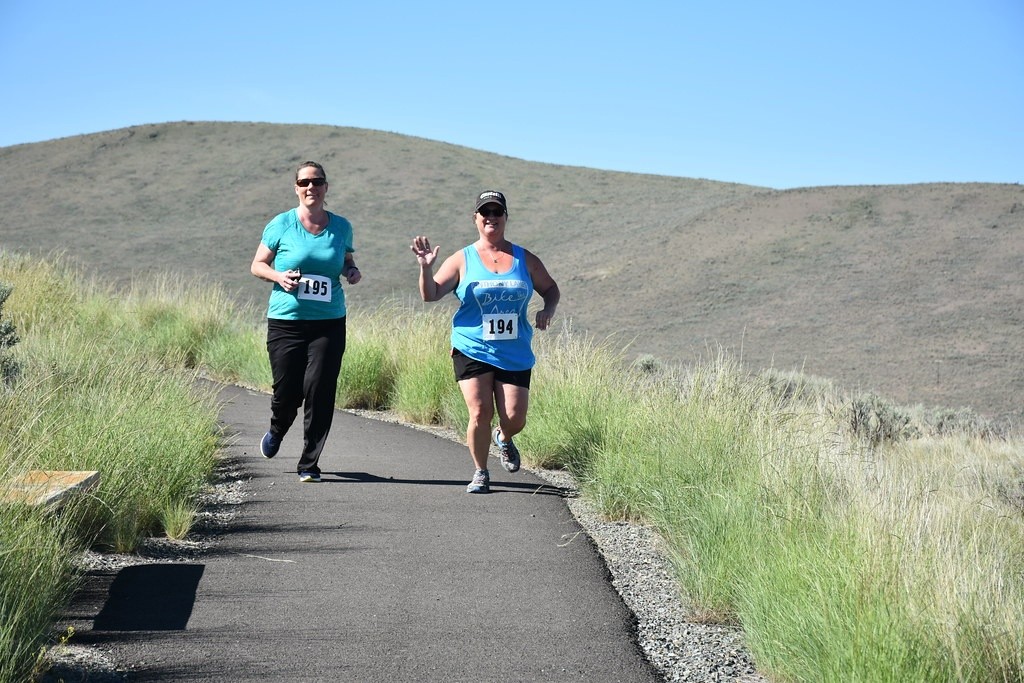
[347,266,358,271]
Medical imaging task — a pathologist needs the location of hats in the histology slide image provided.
[475,190,506,212]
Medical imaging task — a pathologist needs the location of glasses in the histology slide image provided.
[297,177,325,187]
[478,207,504,217]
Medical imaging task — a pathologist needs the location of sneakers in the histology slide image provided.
[299,471,321,482]
[492,426,520,472]
[467,469,490,493]
[260,431,282,458]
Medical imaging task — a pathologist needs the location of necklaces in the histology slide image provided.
[485,246,505,263]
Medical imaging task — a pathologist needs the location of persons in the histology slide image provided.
[409,189,562,494]
[251,161,362,482]
